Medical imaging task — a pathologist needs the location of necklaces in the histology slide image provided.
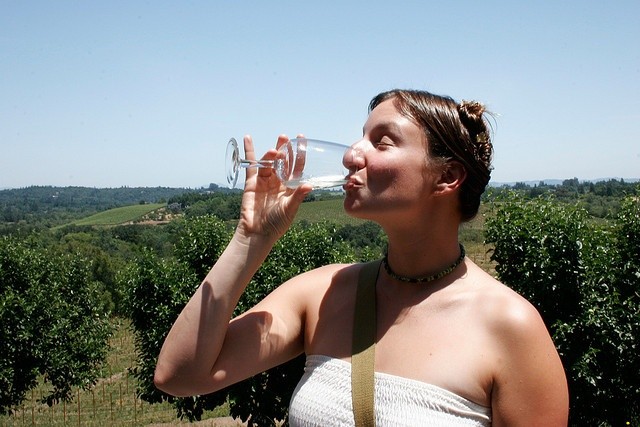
[384,243,465,283]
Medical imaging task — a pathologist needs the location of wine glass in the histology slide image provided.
[224,136,354,190]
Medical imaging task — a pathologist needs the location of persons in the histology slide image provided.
[155,89,570,427]
